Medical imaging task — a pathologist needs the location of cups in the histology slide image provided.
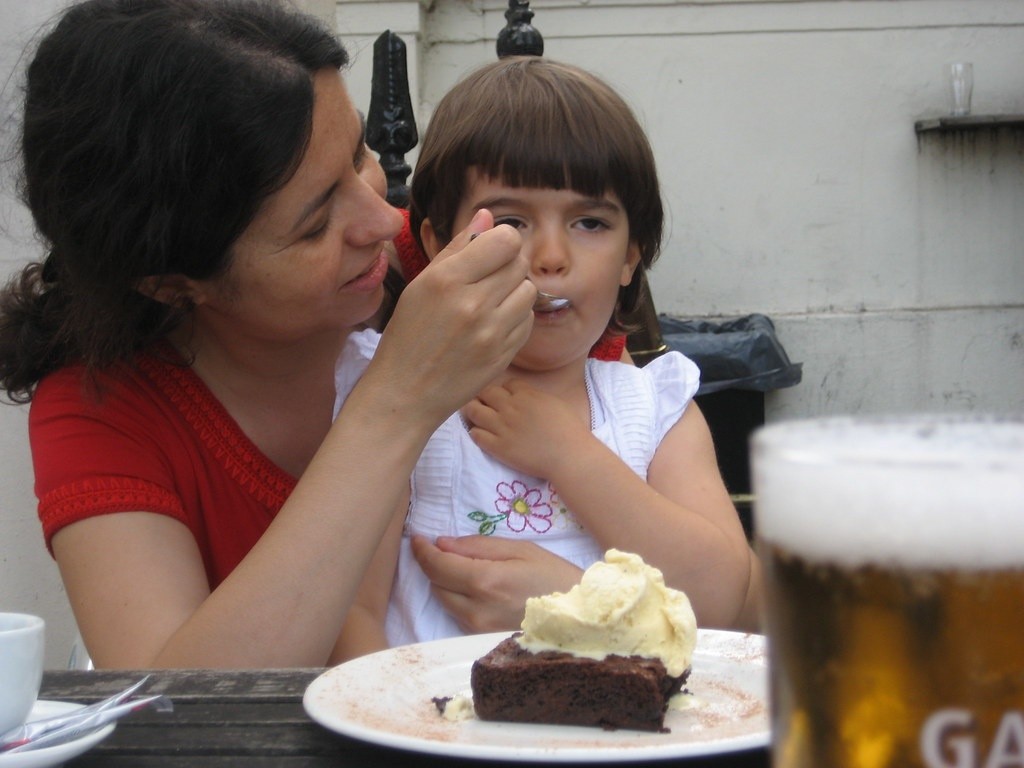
[749,414,1024,768]
[942,62,975,116]
[0,611,51,736]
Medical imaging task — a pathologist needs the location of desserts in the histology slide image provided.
[470,547,697,732]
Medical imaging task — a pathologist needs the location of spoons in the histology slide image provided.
[470,232,571,314]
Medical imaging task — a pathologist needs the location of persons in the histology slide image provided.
[0,1,763,671]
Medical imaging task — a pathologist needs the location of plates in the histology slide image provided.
[0,700,118,768]
[302,624,779,763]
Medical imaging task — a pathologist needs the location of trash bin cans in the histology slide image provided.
[627,310,805,553]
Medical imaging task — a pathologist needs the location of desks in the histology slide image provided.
[38,667,769,768]
